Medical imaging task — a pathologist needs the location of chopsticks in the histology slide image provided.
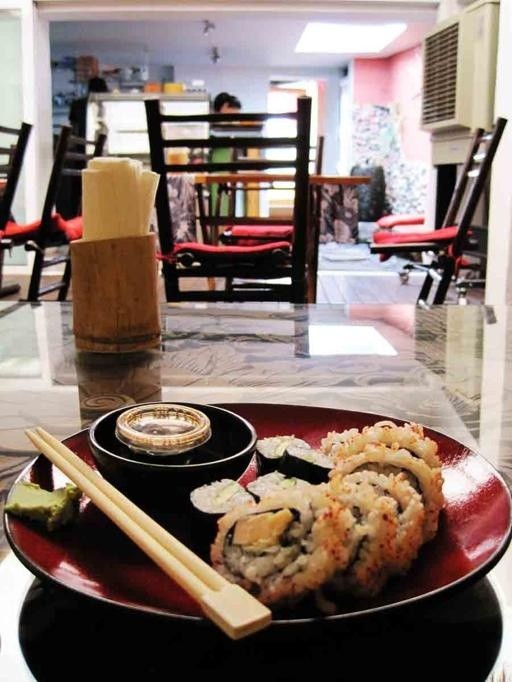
[24,423,275,641]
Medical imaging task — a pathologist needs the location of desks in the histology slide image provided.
[194,175,371,245]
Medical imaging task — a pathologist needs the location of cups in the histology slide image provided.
[66,231,164,355]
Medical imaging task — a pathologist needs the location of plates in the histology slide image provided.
[1,402,512,633]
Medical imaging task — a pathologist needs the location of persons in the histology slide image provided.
[53,74,112,221]
[208,91,243,243]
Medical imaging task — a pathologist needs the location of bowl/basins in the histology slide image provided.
[85,400,262,513]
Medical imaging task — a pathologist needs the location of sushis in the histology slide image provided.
[188,420,444,609]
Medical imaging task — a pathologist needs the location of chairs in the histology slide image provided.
[144,97,312,300]
[1,121,33,266]
[217,224,293,293]
[2,120,108,301]
[371,117,510,310]
